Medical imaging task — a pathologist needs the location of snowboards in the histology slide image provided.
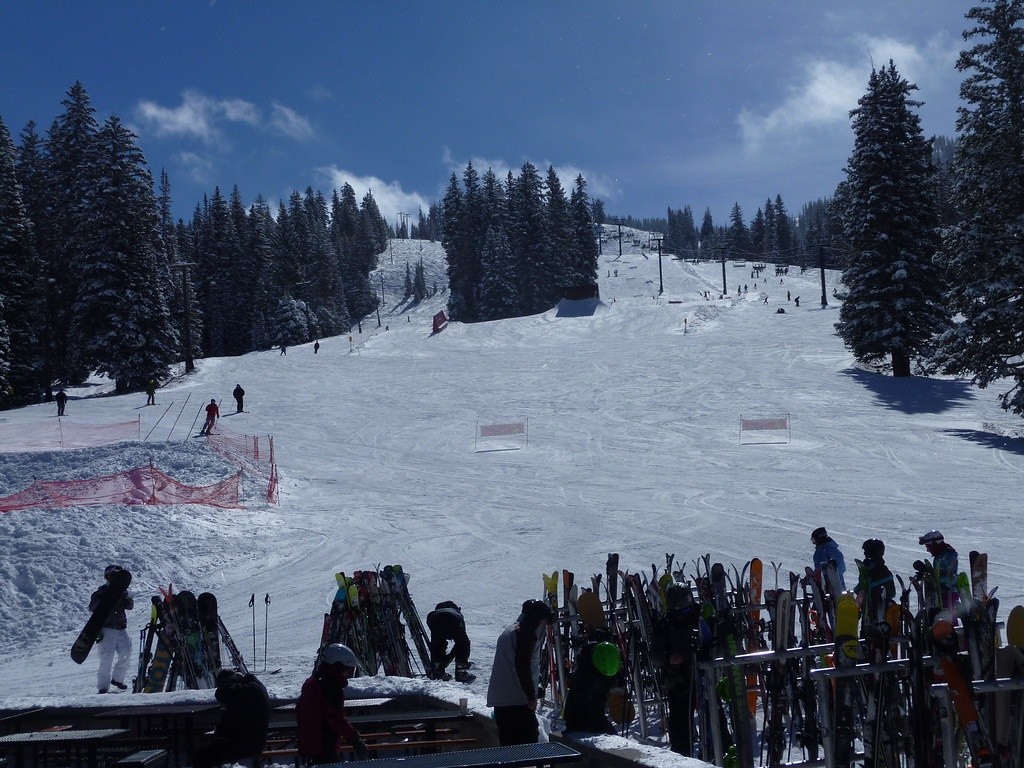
[197,592,222,679]
[176,591,216,689]
[143,594,177,693]
[70,569,132,664]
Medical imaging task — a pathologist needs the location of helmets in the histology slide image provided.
[320,643,361,669]
[862,539,885,558]
[923,531,944,544]
[521,599,551,620]
[591,641,620,677]
[665,582,691,609]
[103,565,122,578]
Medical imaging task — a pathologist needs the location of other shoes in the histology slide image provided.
[111,679,127,689]
[455,669,476,682]
[431,669,451,680]
[99,689,107,693]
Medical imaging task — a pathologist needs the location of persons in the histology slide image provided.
[296,644,368,765]
[233,384,245,412]
[918,531,959,626]
[738,264,837,307]
[56,388,67,416]
[280,346,287,356]
[801,526,847,593]
[89,565,134,693]
[427,607,475,681]
[564,628,622,735]
[653,582,732,762]
[191,668,269,768]
[146,380,155,405]
[202,399,219,434]
[853,538,896,600]
[486,598,552,747]
[314,341,320,354]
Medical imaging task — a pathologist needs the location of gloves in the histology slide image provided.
[346,729,368,760]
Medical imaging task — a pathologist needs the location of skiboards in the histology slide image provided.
[131,582,249,693]
[537,552,1024,768]
[314,563,435,681]
[192,432,220,438]
[248,668,282,675]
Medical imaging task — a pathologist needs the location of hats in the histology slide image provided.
[215,669,233,684]
[812,527,827,543]
